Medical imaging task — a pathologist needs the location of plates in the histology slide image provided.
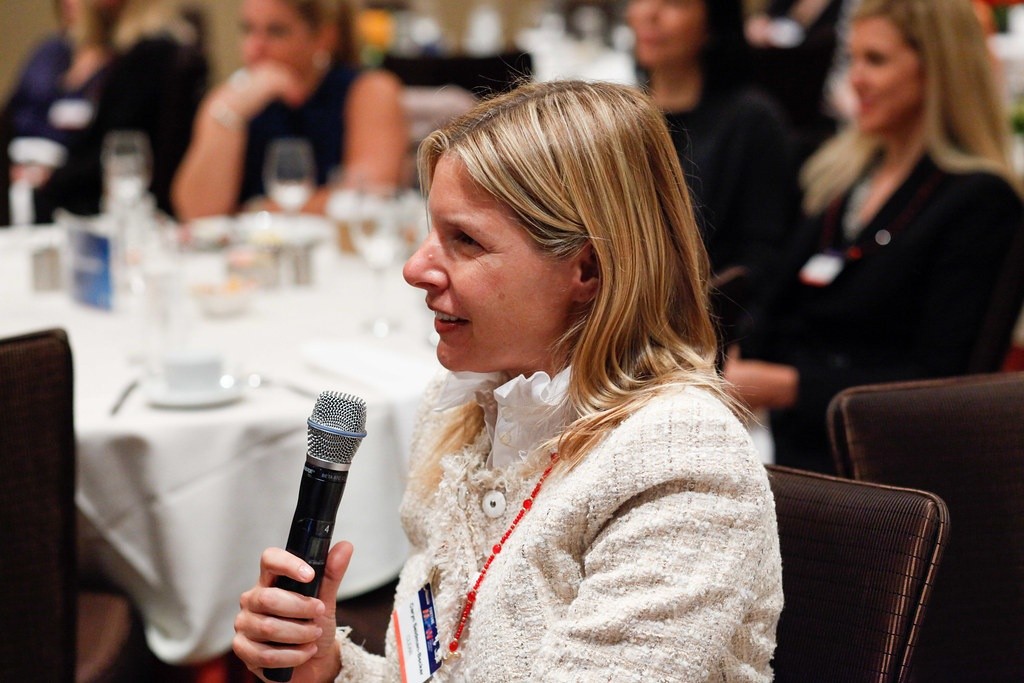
[147,394,239,405]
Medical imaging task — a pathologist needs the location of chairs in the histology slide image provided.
[765,464,953,683]
[825,372,1024,683]
[0,327,129,683]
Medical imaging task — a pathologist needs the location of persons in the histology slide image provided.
[1,0,1024,475]
[230,80,786,683]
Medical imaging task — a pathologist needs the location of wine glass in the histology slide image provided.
[104,130,432,328]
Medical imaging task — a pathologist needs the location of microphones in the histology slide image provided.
[262,392,370,683]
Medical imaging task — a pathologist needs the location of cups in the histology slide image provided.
[164,354,222,404]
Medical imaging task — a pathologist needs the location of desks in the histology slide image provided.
[0,223,450,663]
